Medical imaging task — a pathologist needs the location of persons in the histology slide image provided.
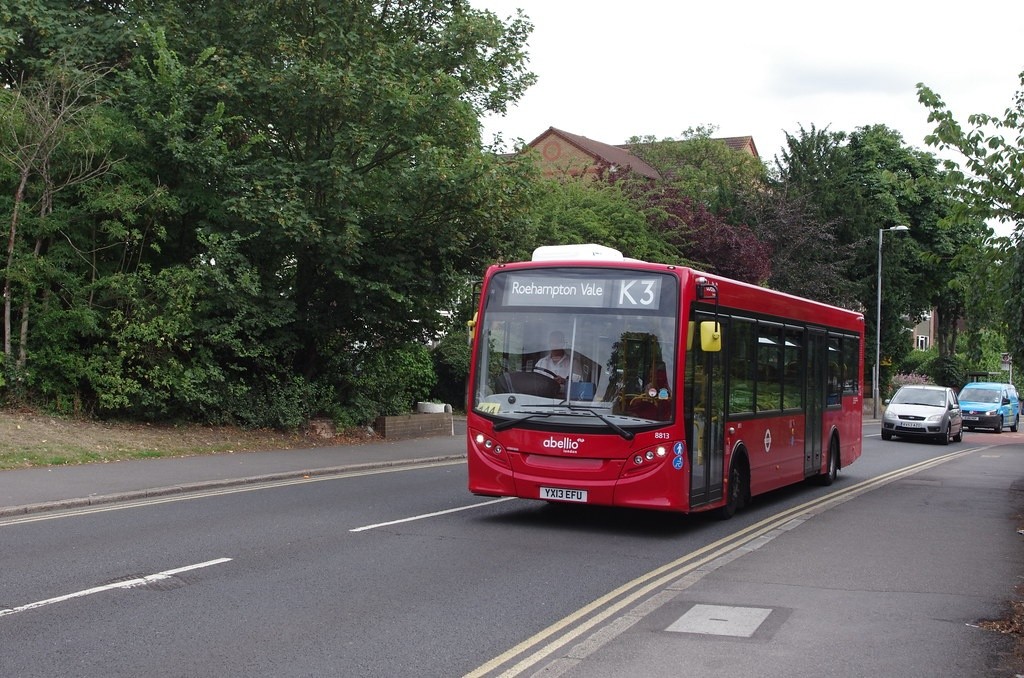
[533,331,583,399]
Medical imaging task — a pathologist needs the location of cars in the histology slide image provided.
[950,381,1020,434]
[880,384,963,445]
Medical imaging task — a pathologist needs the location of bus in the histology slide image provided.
[466,239,866,522]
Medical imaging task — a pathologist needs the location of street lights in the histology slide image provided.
[873,225,912,417]
